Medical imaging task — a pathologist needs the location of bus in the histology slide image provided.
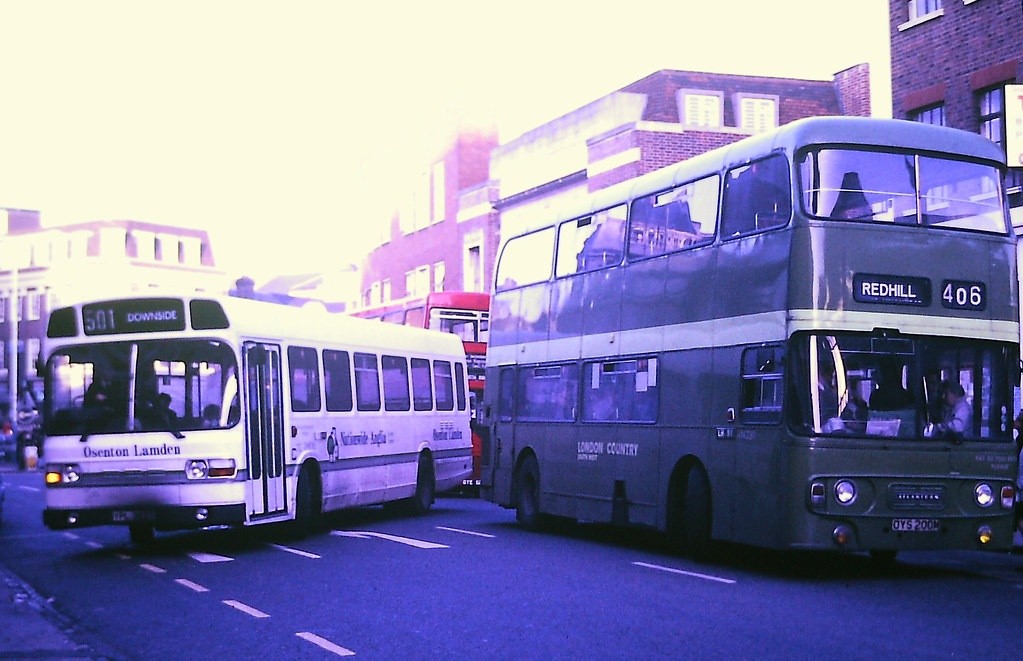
[478,113,1023,563]
[33,288,474,543]
[347,291,491,480]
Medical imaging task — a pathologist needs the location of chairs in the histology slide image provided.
[364,397,452,411]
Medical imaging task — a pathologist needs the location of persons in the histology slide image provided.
[83,364,127,422]
[929,378,972,438]
[869,364,923,415]
[147,391,177,428]
